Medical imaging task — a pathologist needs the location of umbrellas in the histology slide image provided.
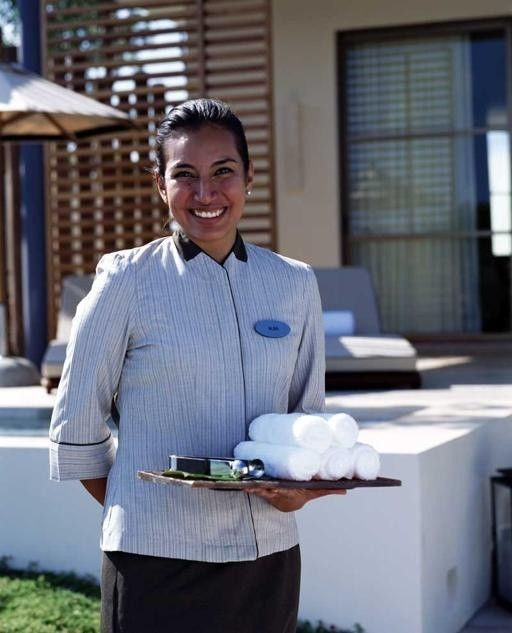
[0,57,142,143]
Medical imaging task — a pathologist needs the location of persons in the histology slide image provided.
[46,95,347,631]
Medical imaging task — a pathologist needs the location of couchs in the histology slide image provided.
[40,268,423,393]
